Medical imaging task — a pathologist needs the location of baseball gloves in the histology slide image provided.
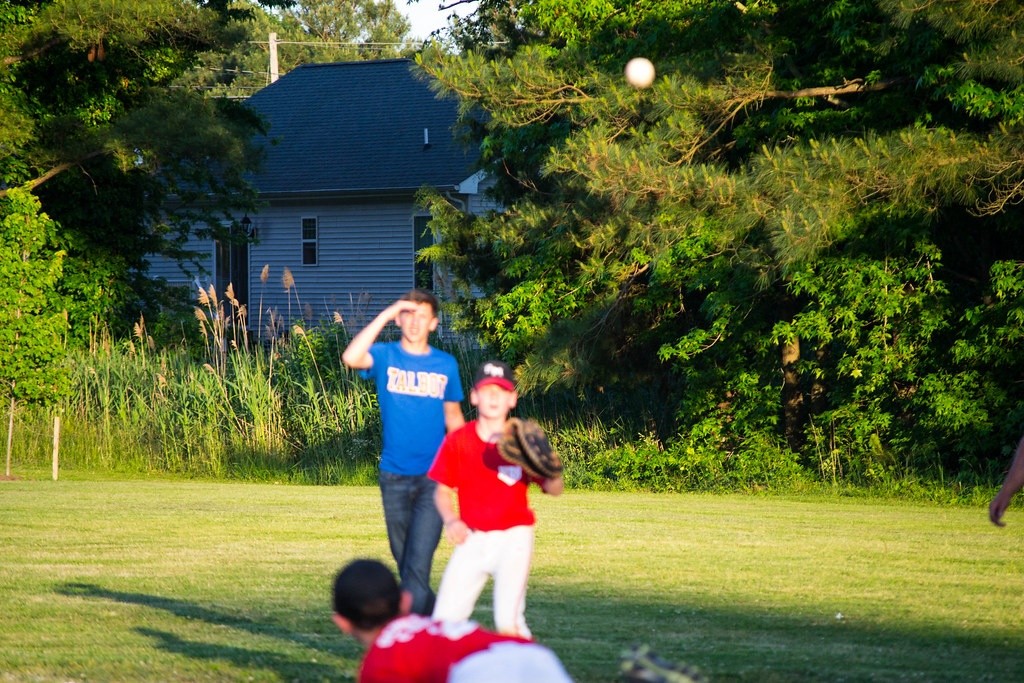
[495,416,564,479]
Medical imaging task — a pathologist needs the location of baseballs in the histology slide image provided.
[624,56,656,89]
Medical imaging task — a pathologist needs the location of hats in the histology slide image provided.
[474,360,514,391]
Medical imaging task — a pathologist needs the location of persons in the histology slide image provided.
[426,360,563,640]
[329,555,574,683]
[989,435,1024,527]
[341,291,465,616]
